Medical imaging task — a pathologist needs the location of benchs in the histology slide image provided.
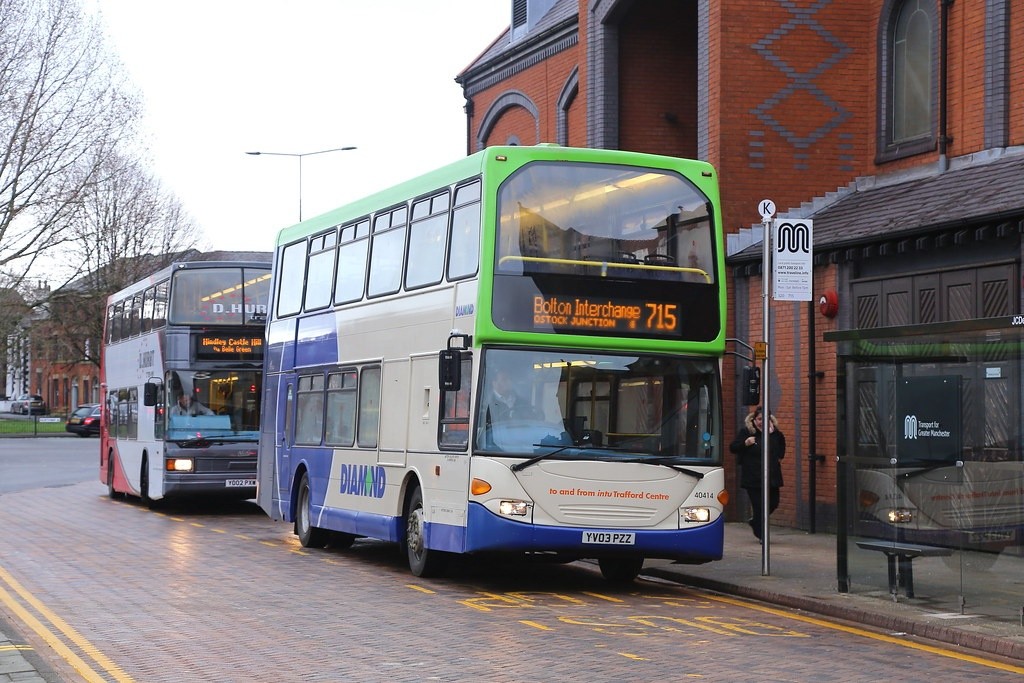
[860,541,954,601]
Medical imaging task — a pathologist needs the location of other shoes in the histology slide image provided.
[747,516,762,544]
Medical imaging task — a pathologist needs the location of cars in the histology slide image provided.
[110,406,138,424]
[65,403,101,438]
[10,394,46,415]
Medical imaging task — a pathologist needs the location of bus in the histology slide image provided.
[98,260,276,510]
[254,139,760,585]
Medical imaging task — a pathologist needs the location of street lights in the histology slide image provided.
[245,144,359,222]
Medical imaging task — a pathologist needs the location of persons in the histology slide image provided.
[480,367,544,427]
[729,405,786,538]
[171,391,215,415]
